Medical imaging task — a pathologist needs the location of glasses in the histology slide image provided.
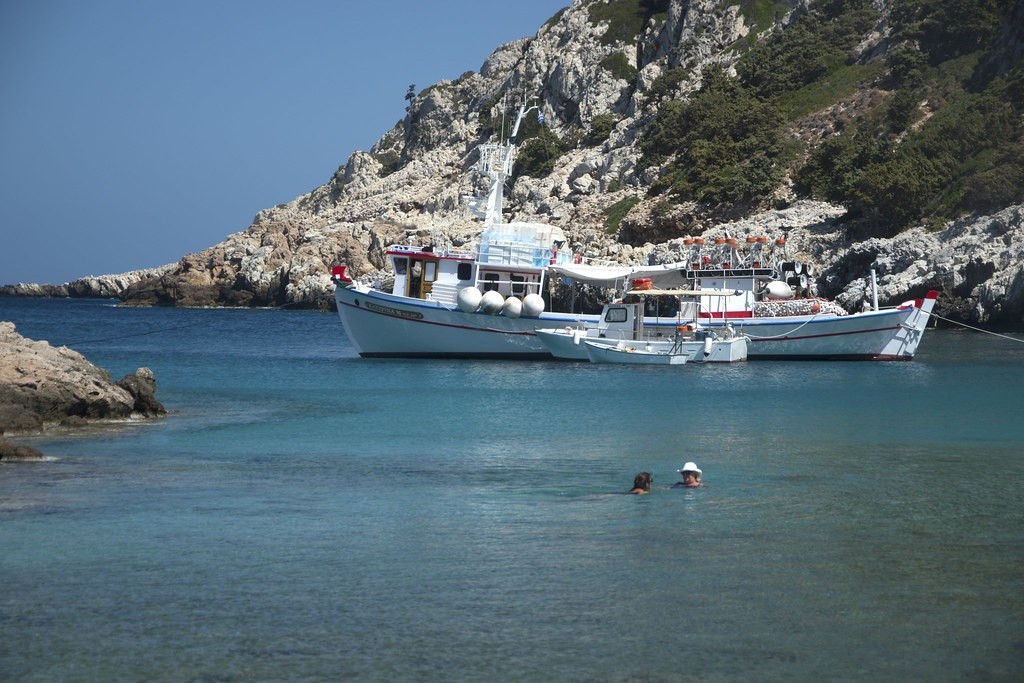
[650,479,653,482]
[681,470,692,475]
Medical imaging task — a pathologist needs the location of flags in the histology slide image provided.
[538,110,544,124]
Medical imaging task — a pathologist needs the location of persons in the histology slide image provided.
[630,472,653,492]
[677,462,704,485]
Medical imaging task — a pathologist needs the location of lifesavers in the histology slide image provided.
[633,277,652,290]
[574,253,581,264]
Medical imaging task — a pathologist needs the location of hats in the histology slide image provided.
[677,462,702,476]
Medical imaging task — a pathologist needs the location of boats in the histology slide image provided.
[330,83,936,357]
[584,323,689,366]
[535,290,749,363]
